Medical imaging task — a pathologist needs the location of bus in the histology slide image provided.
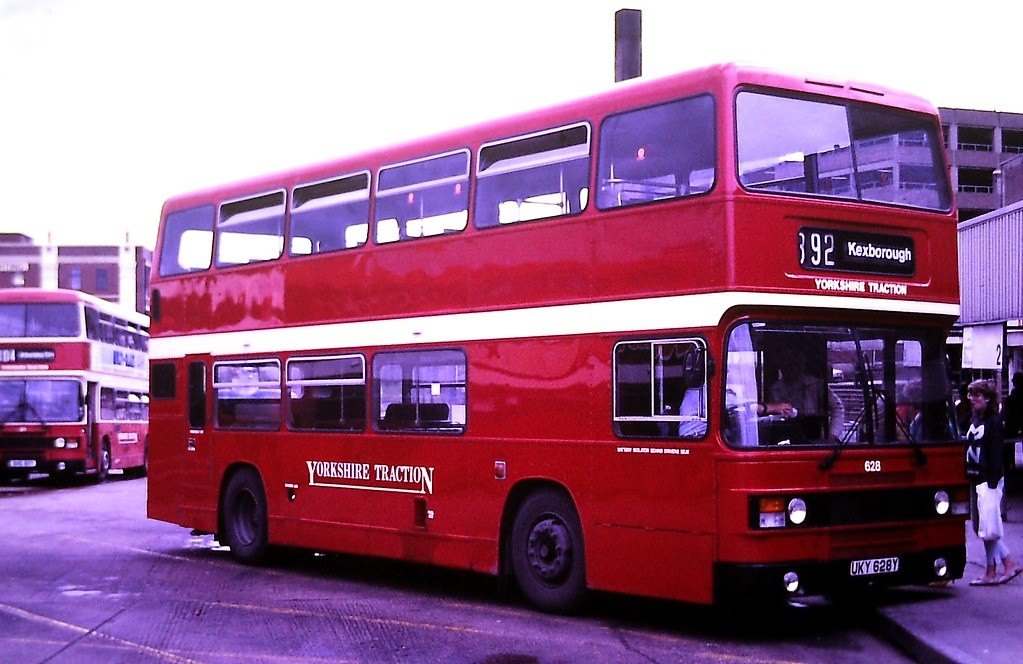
[142,63,971,615]
[0,286,151,487]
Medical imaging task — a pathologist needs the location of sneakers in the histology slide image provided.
[968,574,999,585]
[999,565,1022,583]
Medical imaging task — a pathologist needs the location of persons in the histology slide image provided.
[965,380,1023,587]
[769,350,845,441]
[956,382,973,432]
[1000,372,1023,437]
[904,378,957,443]
[678,366,793,437]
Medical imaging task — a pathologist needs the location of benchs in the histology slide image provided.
[217,396,452,432]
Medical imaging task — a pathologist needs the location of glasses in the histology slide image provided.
[966,392,983,401]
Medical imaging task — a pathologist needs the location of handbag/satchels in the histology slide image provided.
[976,479,1004,542]
